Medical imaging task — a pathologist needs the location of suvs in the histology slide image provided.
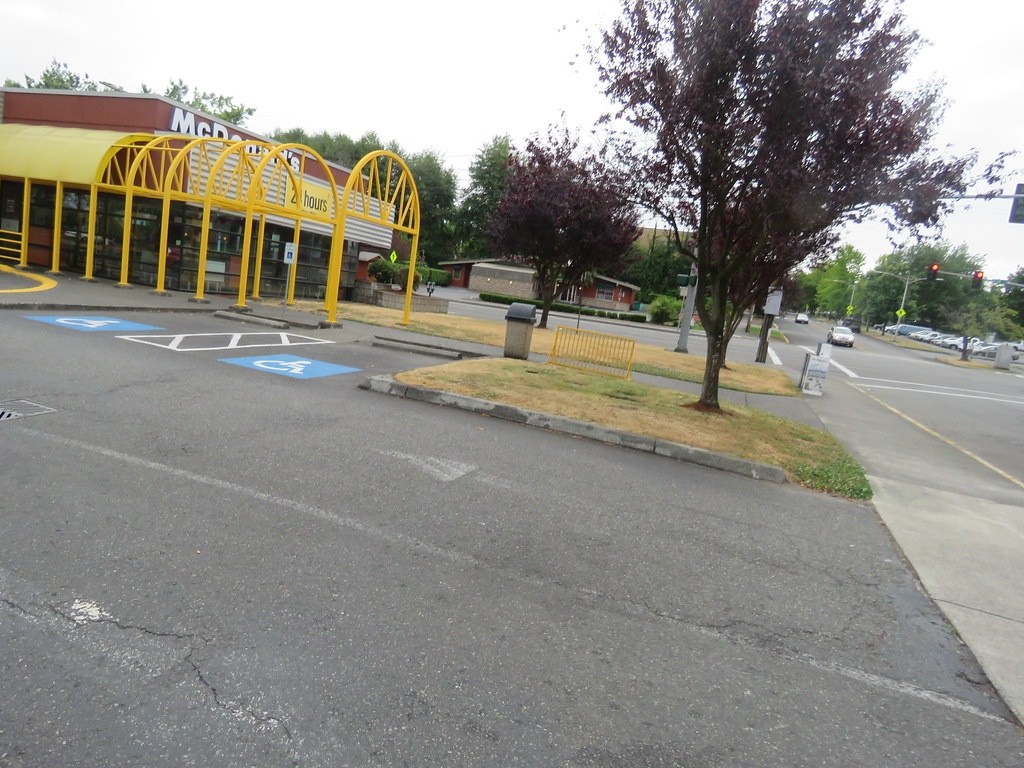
[836,316,861,333]
[939,336,980,351]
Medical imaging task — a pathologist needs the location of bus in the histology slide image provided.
[895,324,932,337]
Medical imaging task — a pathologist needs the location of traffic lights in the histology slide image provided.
[970,271,983,290]
[926,264,939,282]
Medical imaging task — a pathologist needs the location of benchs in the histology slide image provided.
[544,325,635,380]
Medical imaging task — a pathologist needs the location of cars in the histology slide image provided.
[973,345,1020,361]
[909,330,930,340]
[965,341,997,353]
[827,326,856,348]
[884,325,898,333]
[1004,342,1024,352]
[874,321,894,332]
[926,334,955,344]
[794,313,808,324]
[915,332,941,342]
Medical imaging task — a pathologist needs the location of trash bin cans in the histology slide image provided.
[504,302,537,360]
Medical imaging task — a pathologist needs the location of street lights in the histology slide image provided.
[873,270,944,343]
[833,279,855,317]
